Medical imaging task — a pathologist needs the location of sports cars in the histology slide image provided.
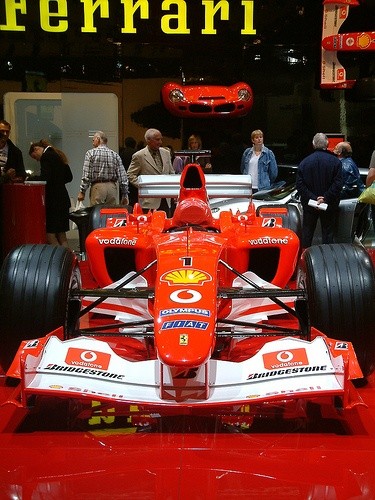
[162,66,254,119]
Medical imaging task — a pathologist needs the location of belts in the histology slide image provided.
[92,179,115,183]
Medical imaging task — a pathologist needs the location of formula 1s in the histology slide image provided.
[1,151,374,430]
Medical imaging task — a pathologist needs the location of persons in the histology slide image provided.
[122,128,214,218]
[333,142,363,198]
[295,133,343,252]
[26,138,73,246]
[77,130,129,205]
[0,120,25,184]
[240,130,278,194]
[366,150,375,232]
